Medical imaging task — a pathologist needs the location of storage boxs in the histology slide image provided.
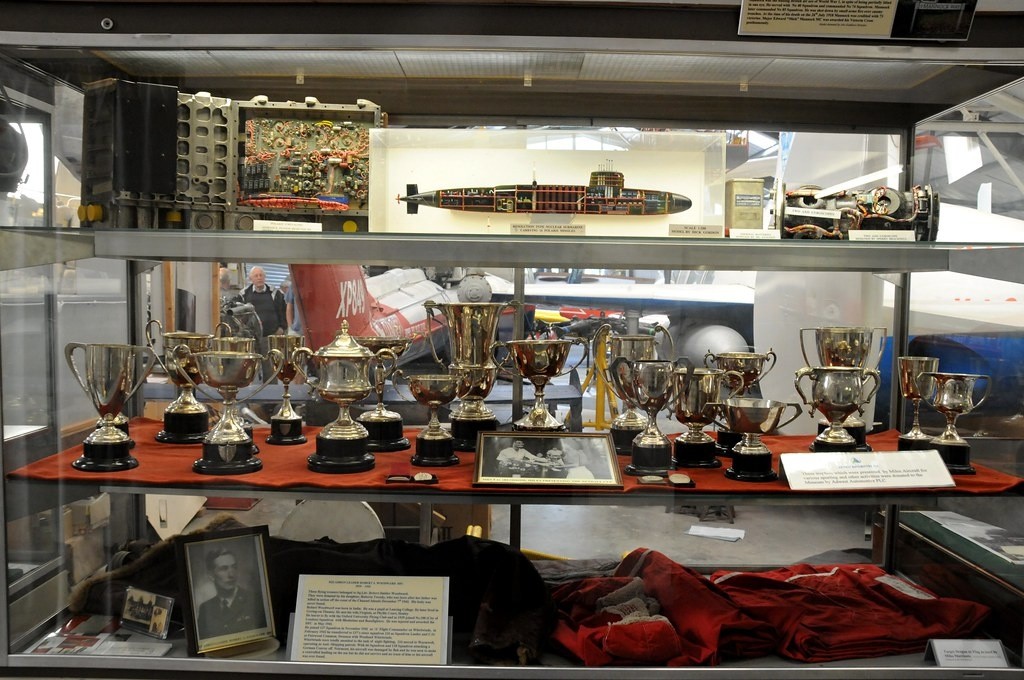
[725,178,765,230]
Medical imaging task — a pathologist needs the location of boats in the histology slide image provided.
[394,172,692,217]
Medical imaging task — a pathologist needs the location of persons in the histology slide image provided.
[548,446,563,466]
[219,266,303,384]
[496,439,547,478]
[198,548,268,640]
[555,440,595,479]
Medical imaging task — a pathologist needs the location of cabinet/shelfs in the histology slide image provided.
[0,0,1024,679]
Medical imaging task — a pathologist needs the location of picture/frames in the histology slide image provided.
[173,524,284,658]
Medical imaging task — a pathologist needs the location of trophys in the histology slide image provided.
[65,302,991,486]
